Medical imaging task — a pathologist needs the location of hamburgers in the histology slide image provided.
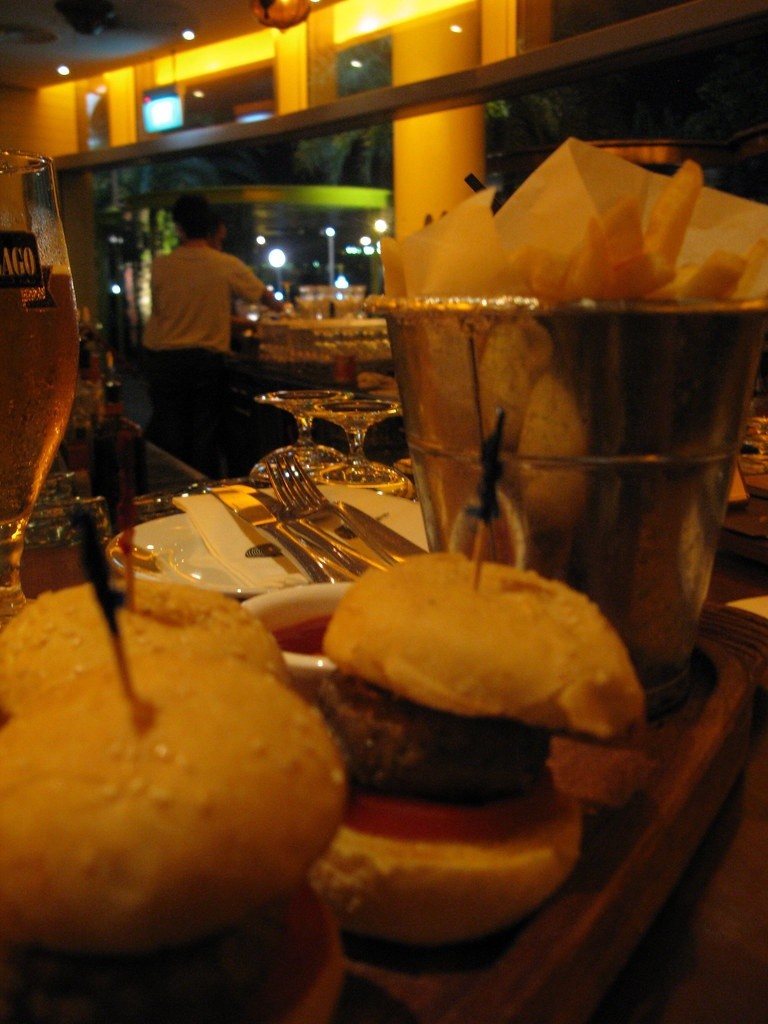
[0,575,349,1024]
[301,552,643,941]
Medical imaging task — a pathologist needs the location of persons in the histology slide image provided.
[143,193,298,481]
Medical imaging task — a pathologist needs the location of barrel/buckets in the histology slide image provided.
[365,292,763,727]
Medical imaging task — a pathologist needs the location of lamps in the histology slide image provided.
[142,82,184,134]
[251,0,312,34]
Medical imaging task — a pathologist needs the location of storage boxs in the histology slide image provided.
[362,294,767,725]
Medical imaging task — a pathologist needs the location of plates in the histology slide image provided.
[104,513,260,595]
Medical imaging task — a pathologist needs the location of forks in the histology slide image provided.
[264,450,398,569]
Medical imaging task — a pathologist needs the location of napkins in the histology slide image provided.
[171,484,406,596]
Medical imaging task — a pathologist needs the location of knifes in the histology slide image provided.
[205,478,331,591]
[337,497,429,559]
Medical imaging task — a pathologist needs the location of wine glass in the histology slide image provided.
[0,132,81,654]
[301,400,417,506]
[252,390,356,487]
